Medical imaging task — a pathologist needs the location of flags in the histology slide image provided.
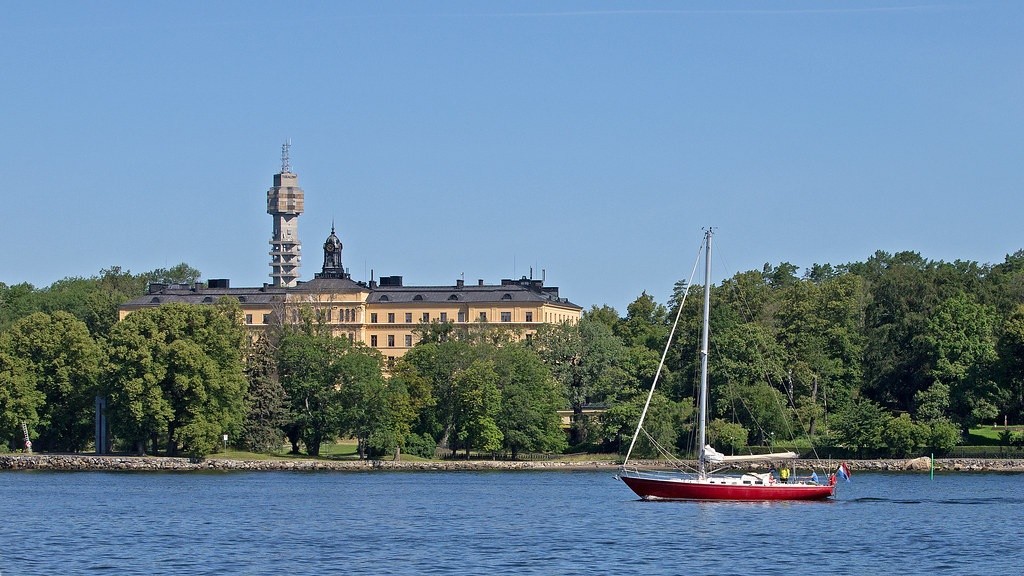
[837,463,852,480]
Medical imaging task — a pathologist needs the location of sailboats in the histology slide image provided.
[613,224,853,500]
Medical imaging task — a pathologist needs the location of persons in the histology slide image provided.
[780,464,790,484]
[808,469,819,483]
